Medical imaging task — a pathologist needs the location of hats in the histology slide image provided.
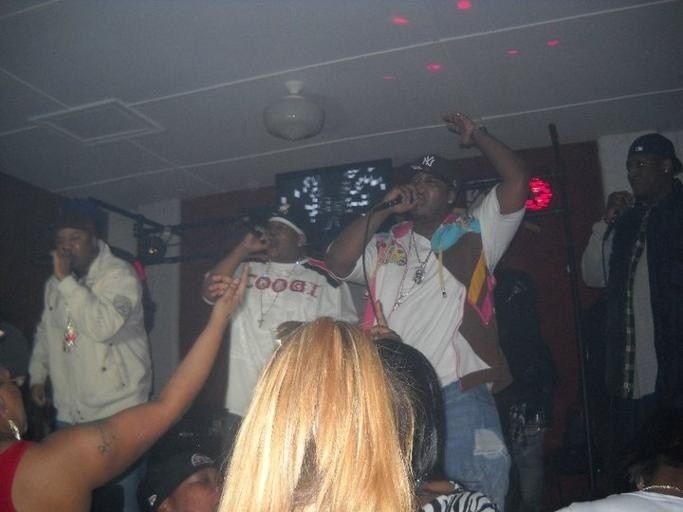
[405,152,460,193]
[0,320,29,376]
[267,203,313,244]
[138,430,218,512]
[627,133,683,178]
[46,198,104,237]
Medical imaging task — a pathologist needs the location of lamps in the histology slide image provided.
[263,81,325,141]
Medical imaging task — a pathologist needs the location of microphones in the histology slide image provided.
[603,211,620,238]
[373,194,405,213]
[242,222,266,244]
